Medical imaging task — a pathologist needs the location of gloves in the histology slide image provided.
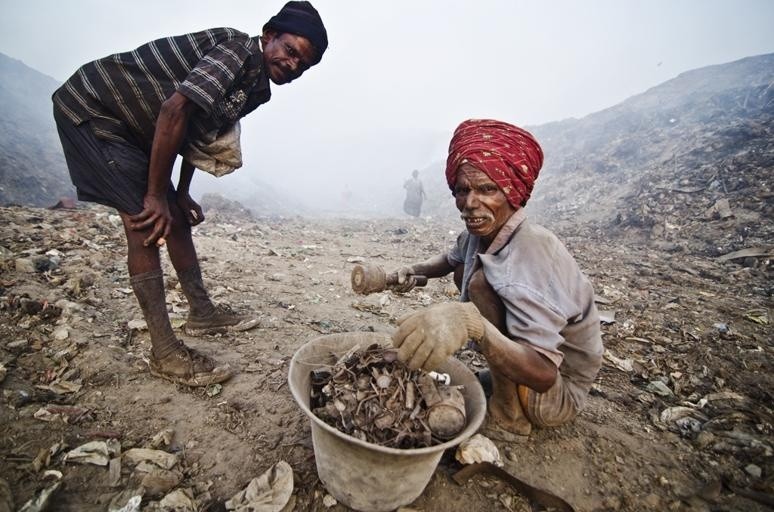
[392,301,486,373]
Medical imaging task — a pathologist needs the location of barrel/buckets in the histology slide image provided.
[288,330,487,510]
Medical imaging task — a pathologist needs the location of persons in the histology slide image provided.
[49,0,329,388]
[404,169,428,219]
[381,118,605,446]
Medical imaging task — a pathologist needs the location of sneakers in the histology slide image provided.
[181,303,261,338]
[148,339,235,388]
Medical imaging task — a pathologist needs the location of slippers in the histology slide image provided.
[478,401,531,445]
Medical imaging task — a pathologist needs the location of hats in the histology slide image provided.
[265,1,330,57]
